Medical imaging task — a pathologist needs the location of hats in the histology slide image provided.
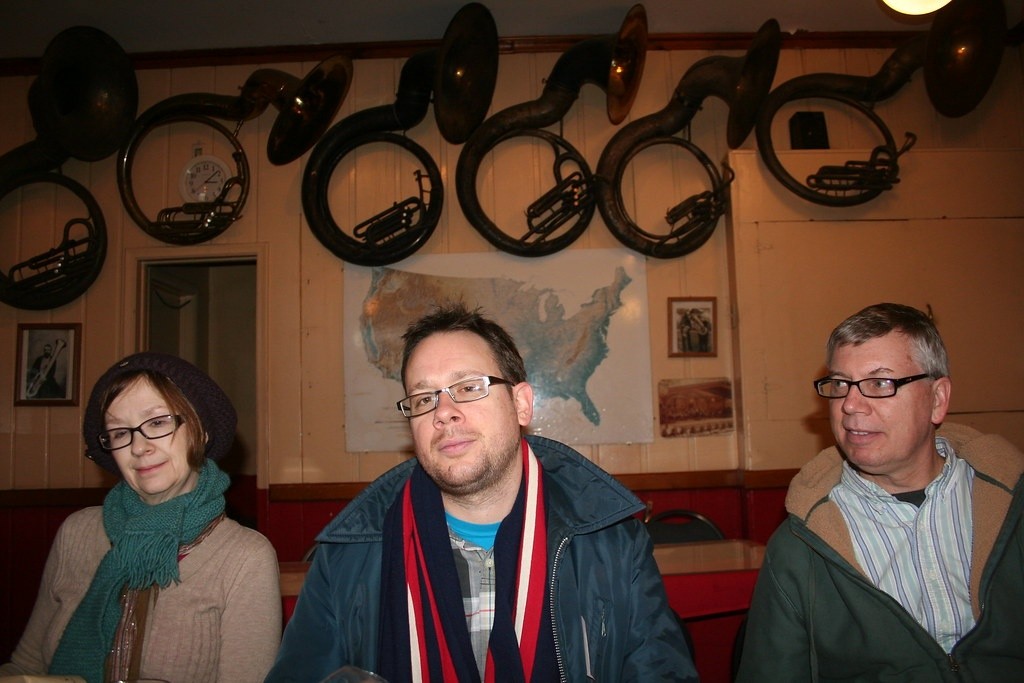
[83,351,236,479]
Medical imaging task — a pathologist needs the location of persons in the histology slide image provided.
[697,320,708,351]
[0,352,283,682]
[32,344,61,397]
[265,294,701,683]
[680,310,693,351]
[733,303,1024,683]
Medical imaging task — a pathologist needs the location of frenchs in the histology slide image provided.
[115,48,356,244]
[595,17,782,261]
[300,2,503,268]
[454,3,651,259]
[1,23,140,314]
[754,2,1004,212]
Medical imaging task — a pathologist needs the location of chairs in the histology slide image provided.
[647,509,726,545]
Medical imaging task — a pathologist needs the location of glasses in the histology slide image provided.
[813,373,931,399]
[397,376,515,418]
[96,414,185,451]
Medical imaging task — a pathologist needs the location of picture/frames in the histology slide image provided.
[667,296,717,358]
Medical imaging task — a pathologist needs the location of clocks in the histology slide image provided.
[178,155,233,203]
[13,322,83,408]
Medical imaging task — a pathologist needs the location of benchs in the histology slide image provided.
[279,539,768,629]
